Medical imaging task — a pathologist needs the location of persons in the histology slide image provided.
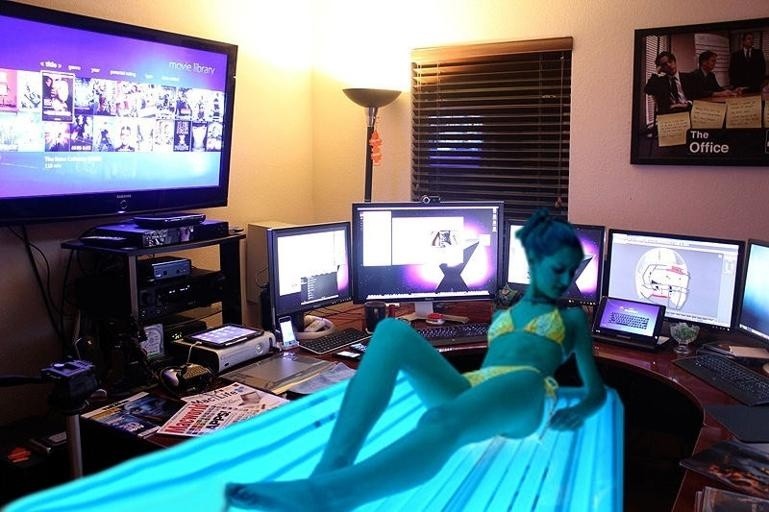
[728,30,768,89]
[42,75,72,111]
[223,204,608,511]
[687,50,739,98]
[642,51,693,115]
[118,125,135,151]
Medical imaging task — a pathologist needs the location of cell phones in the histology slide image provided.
[332,350,361,362]
[278,316,296,346]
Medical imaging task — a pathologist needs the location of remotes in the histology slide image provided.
[80,236,128,249]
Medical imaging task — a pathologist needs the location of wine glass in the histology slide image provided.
[670,323,701,353]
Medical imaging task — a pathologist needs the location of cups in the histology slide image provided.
[363,301,385,332]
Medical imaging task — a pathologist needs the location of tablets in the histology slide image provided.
[183,323,265,350]
[591,295,670,350]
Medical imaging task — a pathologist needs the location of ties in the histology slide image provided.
[671,76,679,101]
[746,49,750,58]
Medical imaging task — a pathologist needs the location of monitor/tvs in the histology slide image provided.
[351,200,504,325]
[266,221,353,341]
[734,238,769,374]
[503,219,605,307]
[602,228,746,333]
[0,0,239,227]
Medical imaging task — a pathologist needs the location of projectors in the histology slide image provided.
[169,330,277,374]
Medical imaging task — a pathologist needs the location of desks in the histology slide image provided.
[80,297,769,512]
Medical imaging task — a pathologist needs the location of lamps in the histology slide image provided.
[343,79,401,202]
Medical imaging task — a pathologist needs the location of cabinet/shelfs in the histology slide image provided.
[59,233,246,333]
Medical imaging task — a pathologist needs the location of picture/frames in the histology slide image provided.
[632,16,768,166]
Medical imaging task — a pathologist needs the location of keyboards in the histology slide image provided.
[672,354,769,407]
[416,322,490,346]
[297,327,372,355]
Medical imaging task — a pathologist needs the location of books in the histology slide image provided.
[179,381,291,413]
[156,401,267,438]
[80,390,188,440]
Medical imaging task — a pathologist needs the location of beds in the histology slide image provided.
[2,364,626,510]
